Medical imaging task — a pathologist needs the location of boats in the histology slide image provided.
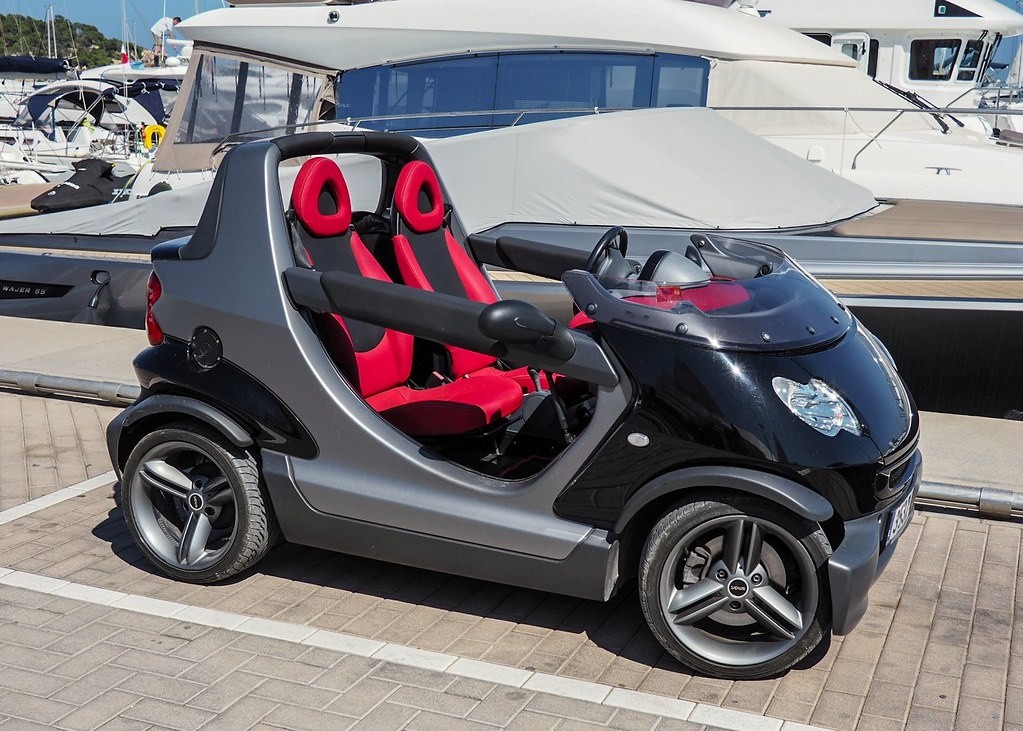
[0,0,1023,418]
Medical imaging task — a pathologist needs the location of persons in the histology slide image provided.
[151,17,182,67]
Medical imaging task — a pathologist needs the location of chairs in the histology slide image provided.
[287,157,522,438]
[390,159,563,393]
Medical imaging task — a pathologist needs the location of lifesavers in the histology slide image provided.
[143,124,167,149]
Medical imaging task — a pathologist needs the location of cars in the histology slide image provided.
[105,129,922,683]
